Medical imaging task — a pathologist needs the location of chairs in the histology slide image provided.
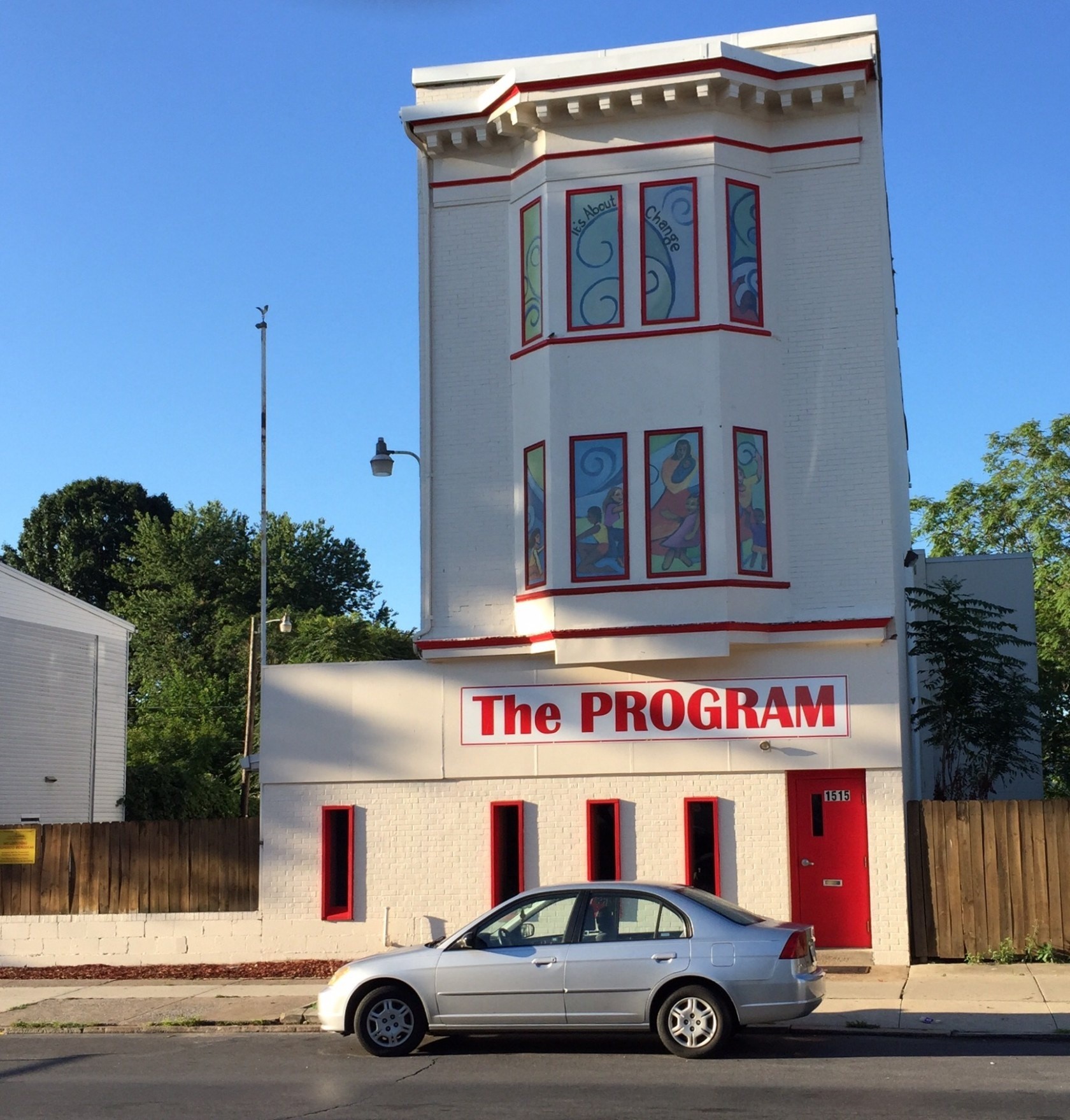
[595,905,615,941]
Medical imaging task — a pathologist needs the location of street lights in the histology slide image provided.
[241,612,292,817]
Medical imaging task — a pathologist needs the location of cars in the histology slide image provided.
[316,882,825,1060]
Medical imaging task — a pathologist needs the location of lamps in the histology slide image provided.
[370,437,421,477]
[45,776,57,782]
[759,739,772,752]
[903,550,919,568]
